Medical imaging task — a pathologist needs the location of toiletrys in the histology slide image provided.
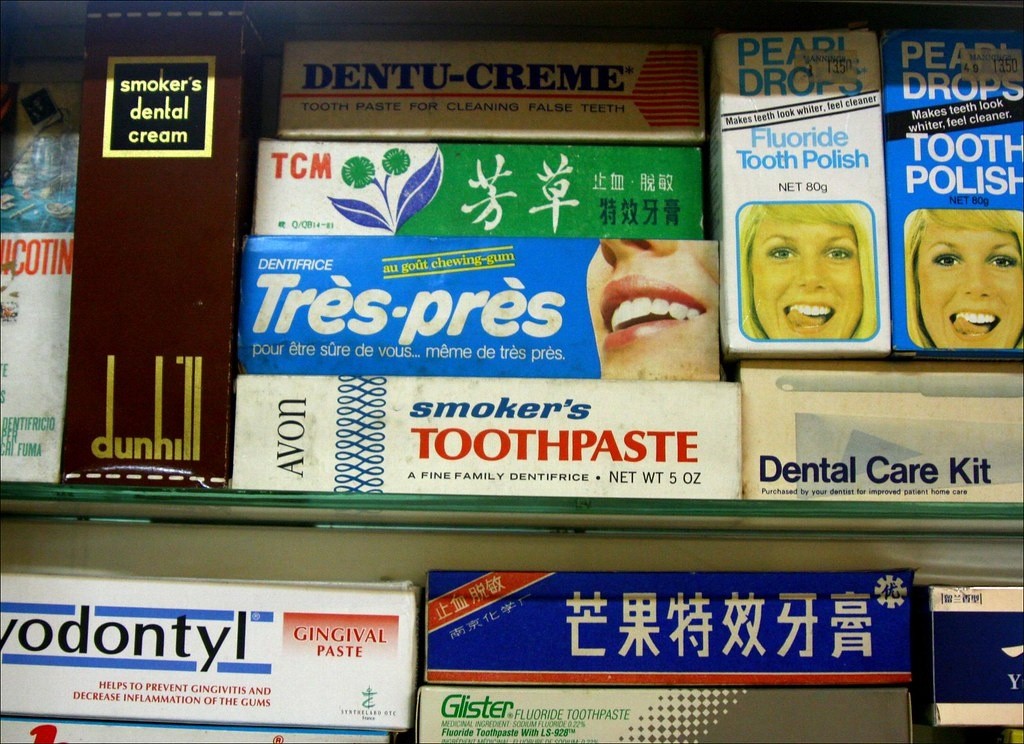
[0,6,1024,744]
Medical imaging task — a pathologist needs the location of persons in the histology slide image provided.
[739,203,877,339]
[586,239,719,382]
[905,209,1024,349]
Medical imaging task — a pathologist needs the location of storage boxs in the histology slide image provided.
[0,30,1023,504]
[0,566,1024,743]
